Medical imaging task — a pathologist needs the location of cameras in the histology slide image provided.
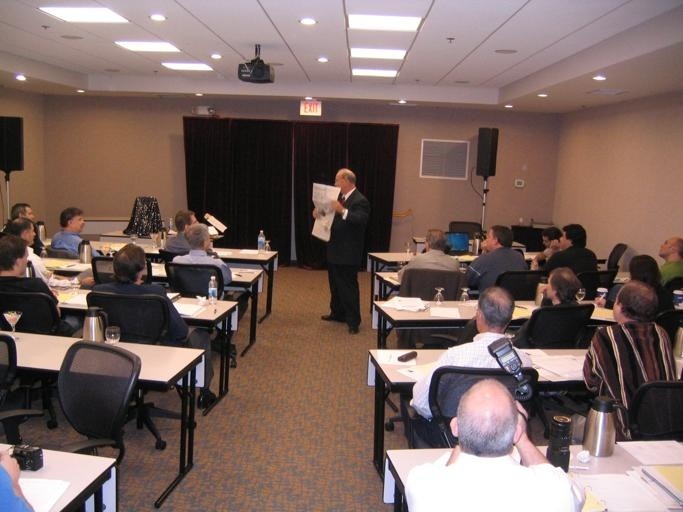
[10,443,43,471]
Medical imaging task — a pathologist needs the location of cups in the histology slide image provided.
[129,233,137,244]
[672,289,682,305]
[596,288,608,299]
[71,276,81,296]
[575,288,585,301]
[104,326,120,344]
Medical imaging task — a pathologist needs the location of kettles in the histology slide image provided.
[534,277,549,306]
[582,396,629,457]
[158,228,166,243]
[81,306,109,344]
[76,239,92,264]
[472,235,484,255]
[34,221,45,241]
[25,261,34,277]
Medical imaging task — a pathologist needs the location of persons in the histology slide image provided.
[93,243,217,409]
[1,451,35,512]
[409,287,534,449]
[52,206,96,277]
[512,267,583,350]
[565,280,677,442]
[162,208,218,258]
[402,378,586,512]
[609,235,682,307]
[1,203,82,336]
[172,222,246,321]
[398,229,459,282]
[312,169,371,335]
[465,225,598,301]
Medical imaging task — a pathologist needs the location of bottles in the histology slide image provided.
[207,275,217,305]
[257,230,265,255]
[546,415,572,472]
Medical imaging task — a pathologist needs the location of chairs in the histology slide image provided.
[448,221,482,240]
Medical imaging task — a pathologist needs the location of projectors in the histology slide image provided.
[238,63,275,84]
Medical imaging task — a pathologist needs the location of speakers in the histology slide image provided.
[476,127,498,176]
[0,116,24,172]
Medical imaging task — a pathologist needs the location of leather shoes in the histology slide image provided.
[321,314,346,323]
[348,324,360,334]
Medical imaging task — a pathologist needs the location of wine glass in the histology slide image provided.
[403,241,412,257]
[432,287,445,306]
[2,310,23,339]
[40,269,53,286]
[458,287,471,307]
[264,241,271,254]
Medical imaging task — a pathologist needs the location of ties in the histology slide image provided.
[339,196,346,206]
[197,391,218,410]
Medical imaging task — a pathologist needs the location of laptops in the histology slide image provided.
[445,231,473,256]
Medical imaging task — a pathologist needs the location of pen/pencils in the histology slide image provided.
[515,306,526,309]
[568,466,589,470]
[595,293,605,304]
